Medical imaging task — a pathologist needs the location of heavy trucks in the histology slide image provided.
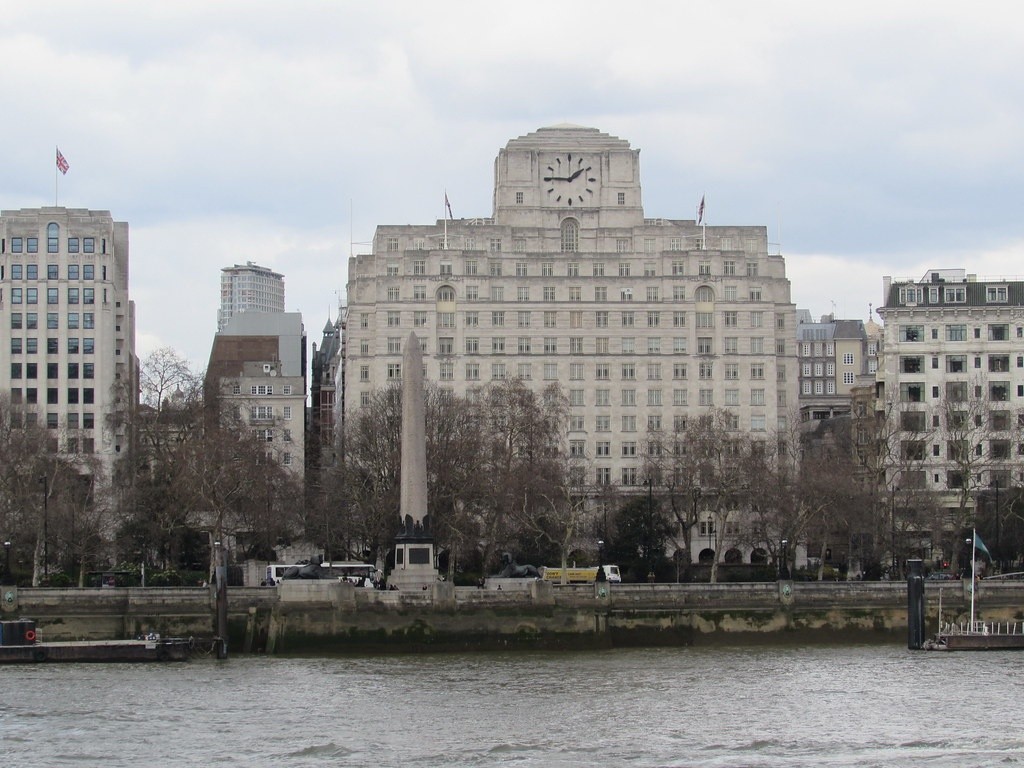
[542,564,621,584]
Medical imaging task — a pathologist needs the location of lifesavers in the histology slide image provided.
[24,630,35,640]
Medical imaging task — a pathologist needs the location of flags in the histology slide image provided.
[698,193,705,226]
[445,192,453,220]
[974,533,995,564]
[56,148,69,175]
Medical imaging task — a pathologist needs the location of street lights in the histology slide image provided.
[778,540,790,580]
[962,538,973,578]
[891,485,901,575]
[642,478,653,554]
[595,540,607,581]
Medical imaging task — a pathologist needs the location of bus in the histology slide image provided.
[261,562,387,591]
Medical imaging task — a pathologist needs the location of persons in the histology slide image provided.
[478,553,1023,590]
[259,573,428,591]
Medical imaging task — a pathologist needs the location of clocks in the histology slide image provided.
[543,154,597,206]
